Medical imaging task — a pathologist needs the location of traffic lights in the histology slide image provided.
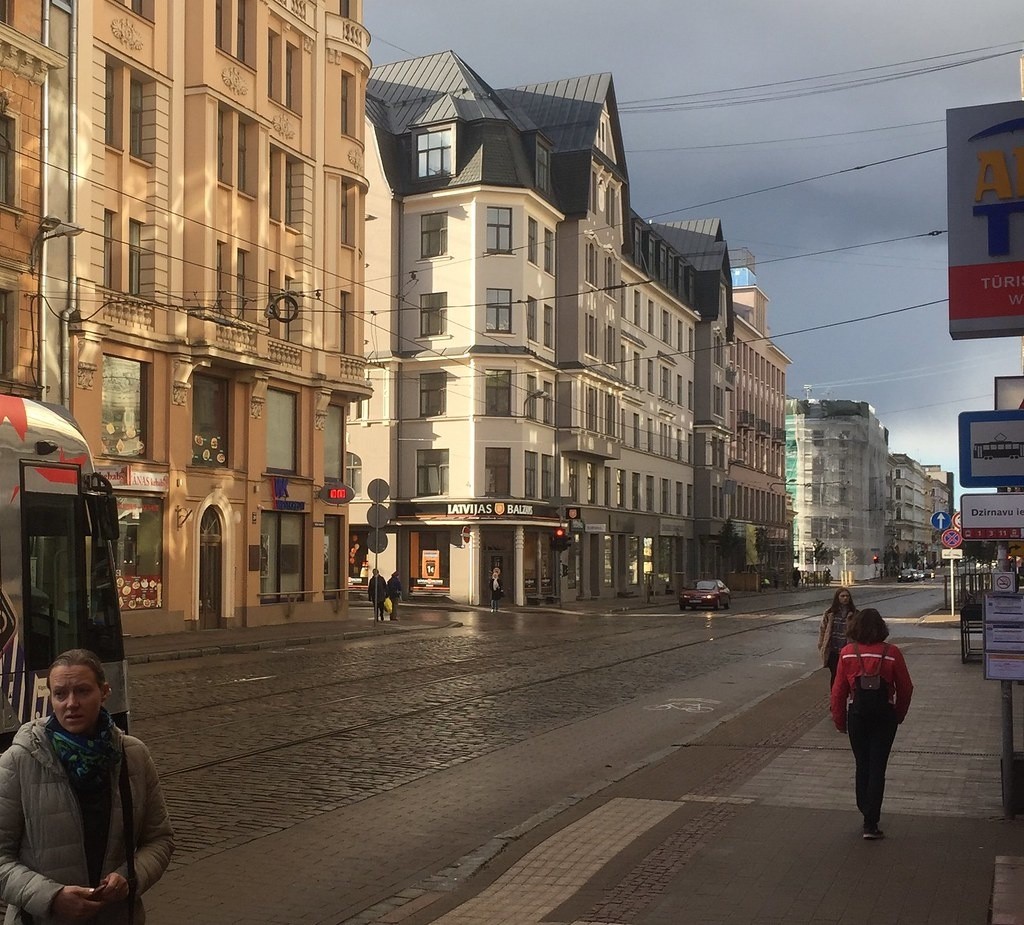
[549,527,572,553]
[873,555,879,563]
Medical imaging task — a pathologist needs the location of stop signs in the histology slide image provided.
[462,526,470,543]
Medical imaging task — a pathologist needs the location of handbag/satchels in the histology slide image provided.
[384,597,393,614]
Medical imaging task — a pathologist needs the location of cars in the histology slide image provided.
[897,568,935,582]
[678,578,731,610]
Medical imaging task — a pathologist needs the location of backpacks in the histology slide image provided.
[853,642,891,723]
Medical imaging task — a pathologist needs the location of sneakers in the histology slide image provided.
[862,822,883,840]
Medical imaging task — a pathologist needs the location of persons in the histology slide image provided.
[367,568,389,623]
[829,608,914,838]
[0,650,173,925]
[388,570,402,621]
[793,560,945,587]
[760,577,770,588]
[490,573,503,613]
[818,587,861,691]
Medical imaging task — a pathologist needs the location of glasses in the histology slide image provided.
[840,595,850,597]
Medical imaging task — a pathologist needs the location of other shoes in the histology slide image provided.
[496,610,499,612]
[491,609,494,612]
[381,619,384,623]
[390,619,398,622]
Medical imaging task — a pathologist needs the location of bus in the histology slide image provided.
[0,392,131,760]
